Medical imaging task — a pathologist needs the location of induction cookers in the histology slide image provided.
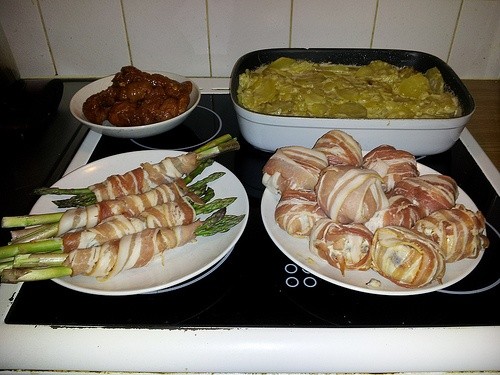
[4,93,500,326]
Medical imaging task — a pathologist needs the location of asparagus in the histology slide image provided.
[0,134,245,284]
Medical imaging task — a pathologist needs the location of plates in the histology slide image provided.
[70,70,201,137]
[261,151,486,296]
[25,150,249,296]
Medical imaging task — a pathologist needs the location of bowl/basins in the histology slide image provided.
[229,46,476,155]
